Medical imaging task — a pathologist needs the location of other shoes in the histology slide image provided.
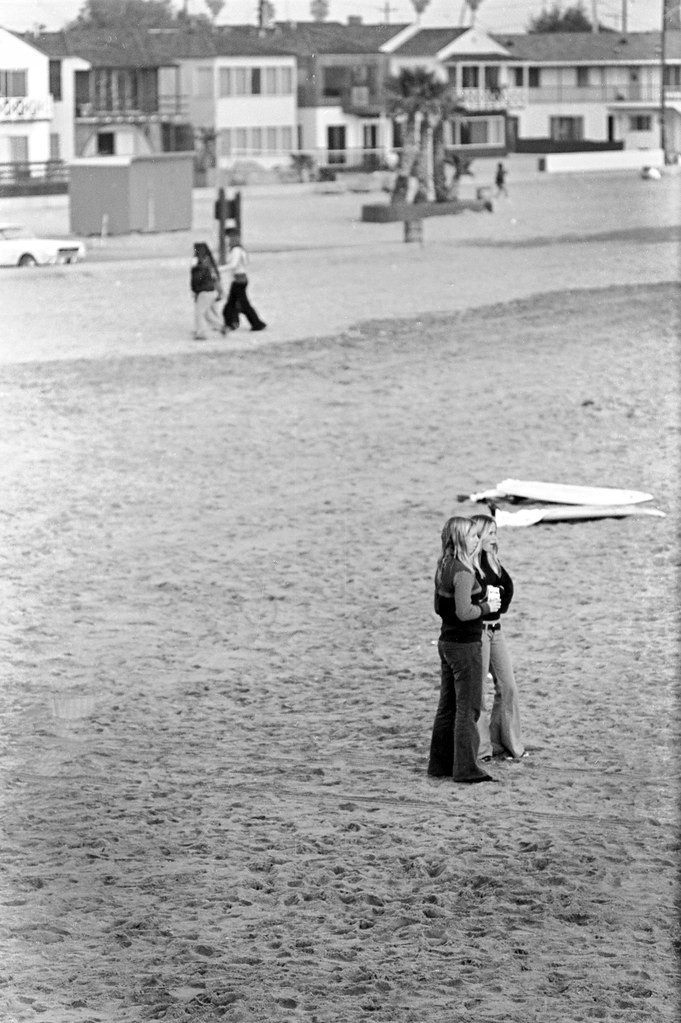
[454,775,498,783]
[481,756,492,763]
[500,750,525,760]
[428,771,453,778]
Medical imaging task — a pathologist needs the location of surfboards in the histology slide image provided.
[489,507,664,529]
[480,480,655,506]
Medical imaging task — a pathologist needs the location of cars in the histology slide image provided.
[0,222,87,268]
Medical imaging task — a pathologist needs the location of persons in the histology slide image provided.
[190,227,268,340]
[427,514,530,783]
[494,163,509,198]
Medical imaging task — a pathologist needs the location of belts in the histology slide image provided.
[482,623,501,632]
